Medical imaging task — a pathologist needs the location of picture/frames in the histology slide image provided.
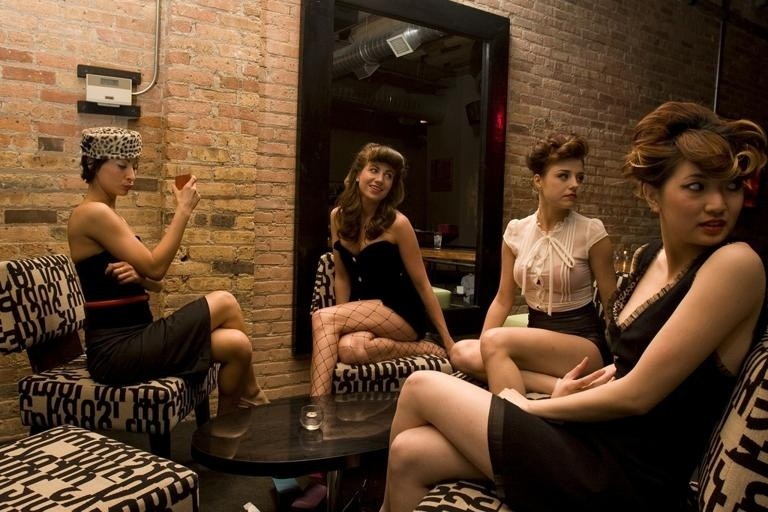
[427,154,455,194]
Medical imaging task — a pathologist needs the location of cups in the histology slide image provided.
[299,403,325,432]
[175,166,191,191]
[433,231,442,250]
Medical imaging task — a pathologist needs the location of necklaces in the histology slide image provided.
[360,224,367,228]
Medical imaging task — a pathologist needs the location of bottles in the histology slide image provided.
[612,249,631,274]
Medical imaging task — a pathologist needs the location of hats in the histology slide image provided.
[81,128,142,160]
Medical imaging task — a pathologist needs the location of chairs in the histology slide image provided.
[0,423,200,512]
[0,252,221,460]
[410,334,767,512]
[448,268,636,392]
[309,250,455,394]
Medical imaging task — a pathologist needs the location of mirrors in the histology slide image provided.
[290,1,511,358]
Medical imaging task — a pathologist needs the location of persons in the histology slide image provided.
[307,142,457,394]
[380,100,767,512]
[68,123,269,416]
[448,128,620,401]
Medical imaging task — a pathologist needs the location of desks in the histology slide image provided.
[420,246,479,318]
